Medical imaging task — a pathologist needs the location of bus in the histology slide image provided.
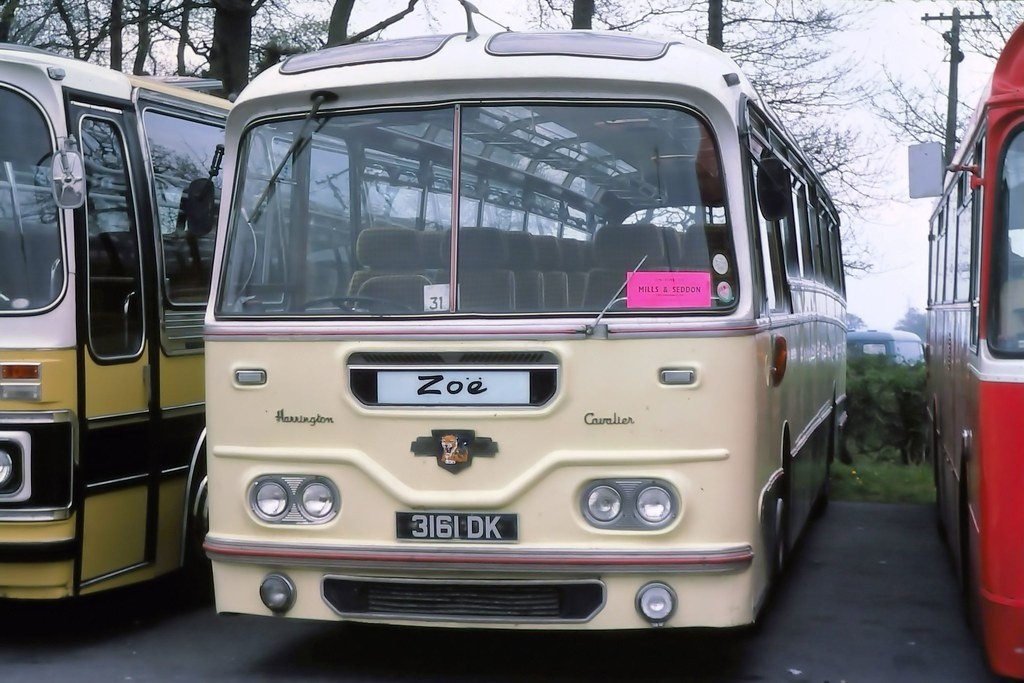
[0,41,599,619]
[924,23,1024,682]
[202,32,844,628]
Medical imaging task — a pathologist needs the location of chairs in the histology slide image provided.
[446,227,515,313]
[534,236,569,311]
[559,238,583,310]
[508,231,545,313]
[684,224,736,305]
[347,228,424,305]
[584,224,668,310]
[357,274,433,316]
[419,231,443,268]
[658,226,681,271]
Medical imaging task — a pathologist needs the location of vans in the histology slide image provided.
[846,328,925,367]
